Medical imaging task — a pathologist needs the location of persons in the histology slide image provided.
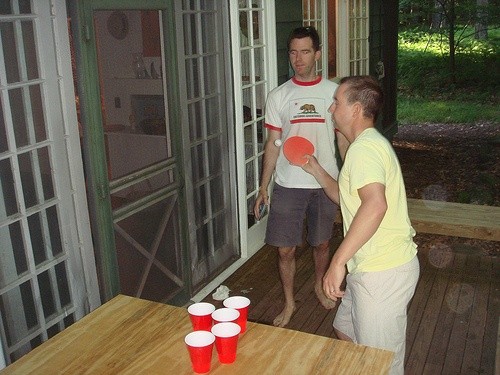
[254,26,350,328]
[288,75,420,374]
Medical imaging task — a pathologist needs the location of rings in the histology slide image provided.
[329,288,335,292]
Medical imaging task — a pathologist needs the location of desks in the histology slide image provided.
[0,293,395,375]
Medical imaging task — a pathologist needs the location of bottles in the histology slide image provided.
[133,53,147,78]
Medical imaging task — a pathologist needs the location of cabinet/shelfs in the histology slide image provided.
[105,135,262,214]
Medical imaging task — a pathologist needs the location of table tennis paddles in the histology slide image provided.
[282,136,315,166]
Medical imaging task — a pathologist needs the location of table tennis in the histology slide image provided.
[274,139,282,147]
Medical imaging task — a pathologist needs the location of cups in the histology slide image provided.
[187,301,216,332]
[114,97,120,107]
[210,308,241,325]
[185,330,216,373]
[223,296,251,334]
[211,322,241,364]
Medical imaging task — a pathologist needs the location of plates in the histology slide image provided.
[239,4,259,39]
[107,10,129,39]
